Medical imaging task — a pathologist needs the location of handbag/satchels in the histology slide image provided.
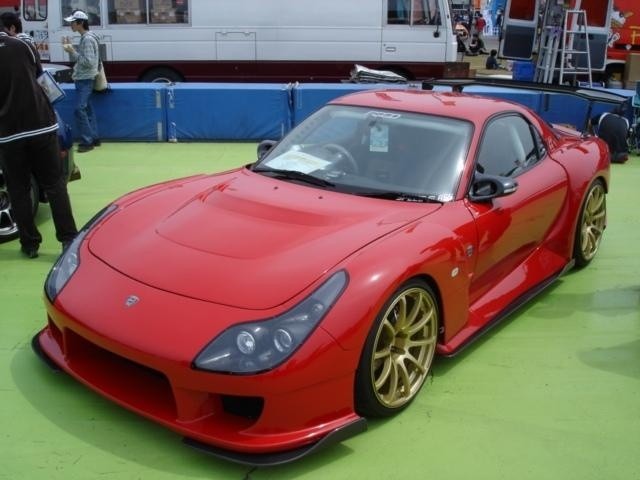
[94,62,109,92]
[36,70,66,107]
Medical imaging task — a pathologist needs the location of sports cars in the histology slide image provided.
[32,79,611,467]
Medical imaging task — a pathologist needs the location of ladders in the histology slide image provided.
[533,0,593,88]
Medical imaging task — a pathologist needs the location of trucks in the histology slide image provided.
[498,0,639,81]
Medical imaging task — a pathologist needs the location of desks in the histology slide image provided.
[437,83,637,143]
[167,83,294,143]
[55,83,166,143]
[294,83,437,143]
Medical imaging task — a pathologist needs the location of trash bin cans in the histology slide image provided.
[511,61,536,81]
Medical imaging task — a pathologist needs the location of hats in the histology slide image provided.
[63,9,89,24]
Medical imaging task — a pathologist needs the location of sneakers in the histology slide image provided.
[77,138,100,153]
[71,165,81,182]
[19,241,39,259]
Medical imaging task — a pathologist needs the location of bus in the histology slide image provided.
[20,0,458,83]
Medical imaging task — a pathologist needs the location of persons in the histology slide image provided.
[589,107,631,163]
[0,12,81,182]
[61,10,101,153]
[0,25,79,260]
[452,0,507,70]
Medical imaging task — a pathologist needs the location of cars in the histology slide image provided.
[0,107,80,241]
